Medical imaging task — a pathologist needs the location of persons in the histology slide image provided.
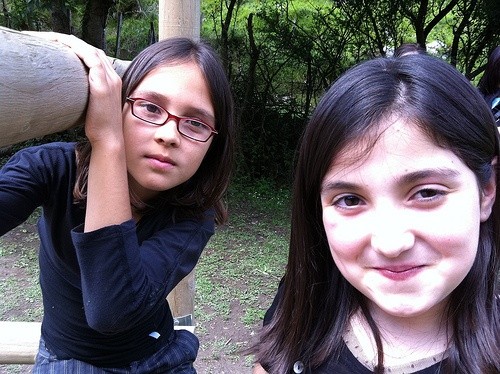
[239,33,500,374]
[0,37,233,374]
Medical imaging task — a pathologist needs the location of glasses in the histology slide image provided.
[125,97,219,142]
